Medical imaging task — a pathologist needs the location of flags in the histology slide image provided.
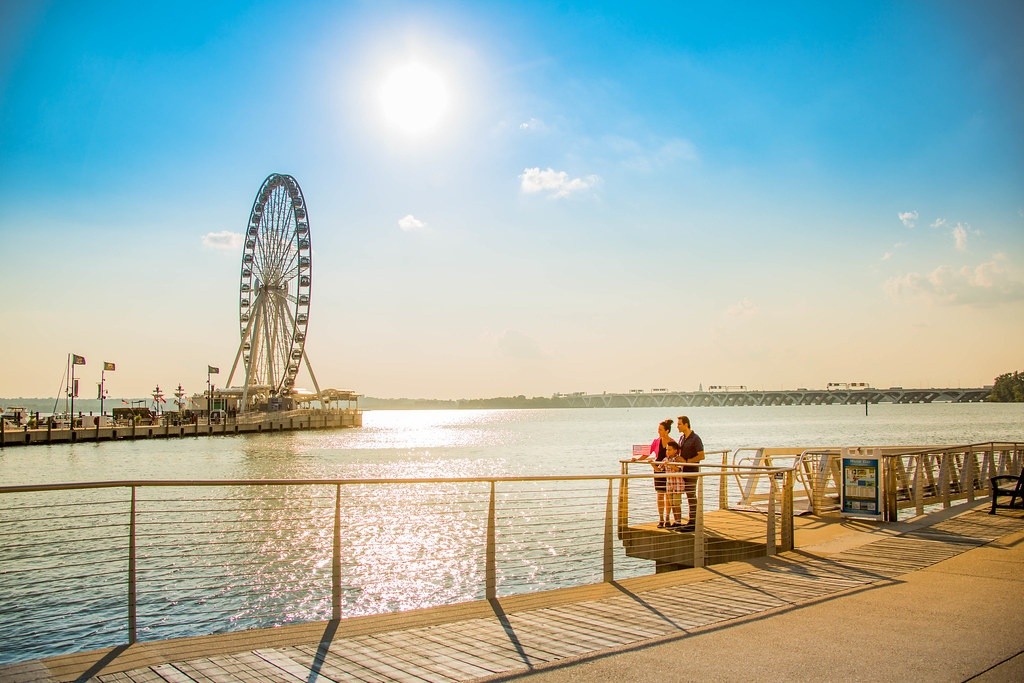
[633,445,651,455]
[208,366,219,374]
[73,354,86,365]
[104,362,115,371]
[121,399,129,404]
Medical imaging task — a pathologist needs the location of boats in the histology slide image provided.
[1,408,29,419]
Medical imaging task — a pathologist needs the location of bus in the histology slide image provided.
[889,387,902,390]
[863,388,875,392]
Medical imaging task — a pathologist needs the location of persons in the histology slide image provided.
[675,416,705,532]
[631,420,680,528]
[648,441,686,529]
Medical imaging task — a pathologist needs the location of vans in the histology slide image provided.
[797,388,808,391]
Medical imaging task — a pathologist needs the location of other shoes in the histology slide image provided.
[657,521,671,528]
[674,524,695,532]
[666,522,685,530]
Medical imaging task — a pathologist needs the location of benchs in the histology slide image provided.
[988,467,1024,515]
